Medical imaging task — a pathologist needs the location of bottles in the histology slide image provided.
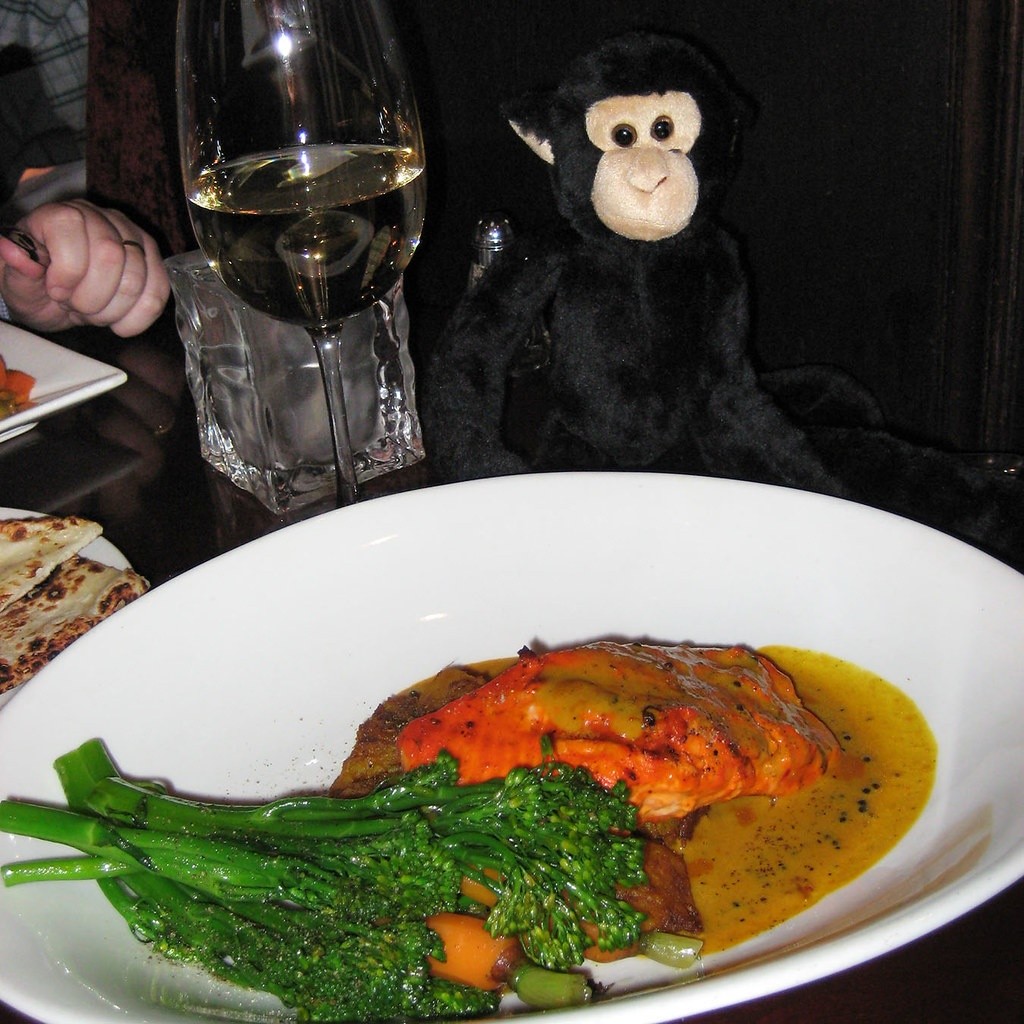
[466,214,551,376]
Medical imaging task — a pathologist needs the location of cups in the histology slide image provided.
[151,225,430,521]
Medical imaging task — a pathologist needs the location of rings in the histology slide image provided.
[123,238,146,253]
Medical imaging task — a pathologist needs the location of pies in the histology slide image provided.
[0,514,151,697]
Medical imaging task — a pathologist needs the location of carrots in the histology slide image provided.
[0,355,36,422]
[411,914,517,993]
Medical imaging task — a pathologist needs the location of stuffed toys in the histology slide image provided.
[418,30,1024,576]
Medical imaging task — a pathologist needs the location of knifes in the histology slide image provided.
[0,216,52,269]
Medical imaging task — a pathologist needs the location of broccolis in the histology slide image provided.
[1,736,647,1024]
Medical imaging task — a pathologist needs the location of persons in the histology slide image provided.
[0,1,171,338]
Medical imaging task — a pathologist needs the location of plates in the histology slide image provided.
[0,321,127,444]
[0,507,140,711]
[0,469,1024,1024]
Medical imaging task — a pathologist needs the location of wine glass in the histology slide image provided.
[175,0,425,509]
